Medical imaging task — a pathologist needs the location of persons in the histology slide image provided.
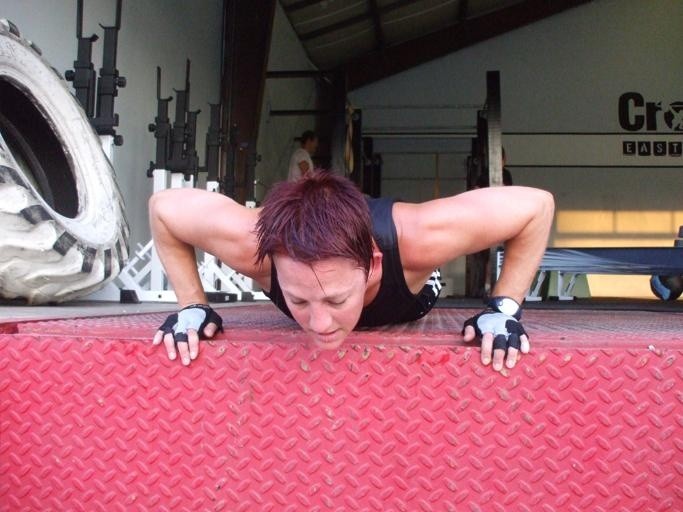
[287,130,317,182]
[145,170,556,370]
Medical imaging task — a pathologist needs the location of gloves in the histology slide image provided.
[157,303,222,343]
[461,294,529,350]
